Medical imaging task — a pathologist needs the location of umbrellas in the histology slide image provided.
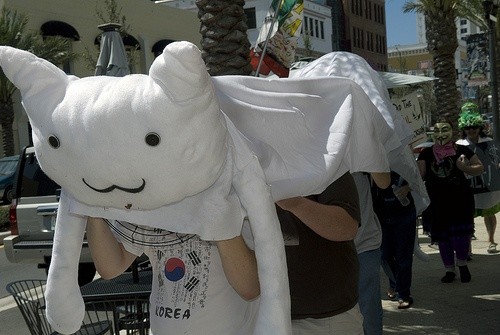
[94,22,130,78]
[290,56,439,89]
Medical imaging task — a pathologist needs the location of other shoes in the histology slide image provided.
[441,272,457,283]
[459,266,472,283]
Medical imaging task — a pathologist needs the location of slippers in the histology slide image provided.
[387,286,397,298]
[486,243,496,253]
[397,296,415,309]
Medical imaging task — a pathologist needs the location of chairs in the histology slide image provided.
[6,279,113,335]
[114,300,151,335]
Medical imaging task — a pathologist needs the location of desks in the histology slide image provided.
[80,271,152,335]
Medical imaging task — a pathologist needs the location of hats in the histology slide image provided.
[457,102,486,129]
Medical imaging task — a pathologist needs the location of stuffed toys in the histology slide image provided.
[0,42,430,335]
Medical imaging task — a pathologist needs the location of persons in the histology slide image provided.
[417,115,484,283]
[87,217,261,335]
[363,171,418,309]
[455,102,500,253]
[276,171,366,335]
[351,171,391,335]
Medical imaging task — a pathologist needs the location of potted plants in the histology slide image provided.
[458,102,487,145]
